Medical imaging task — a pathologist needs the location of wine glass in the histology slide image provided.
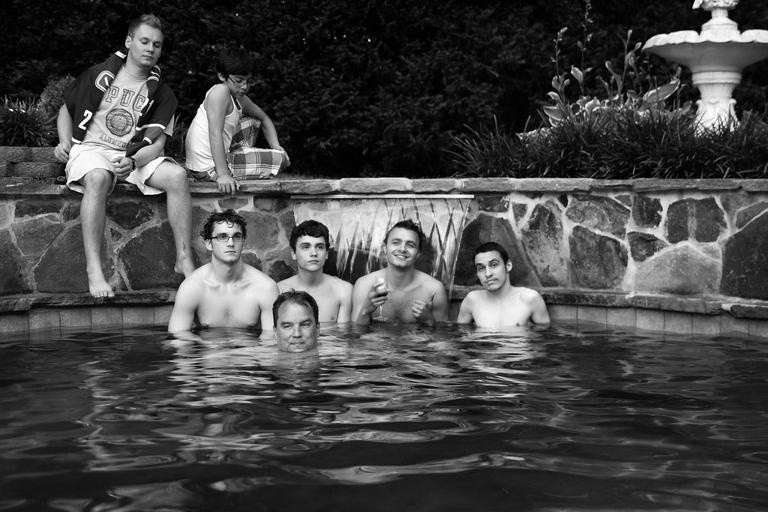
[373,278,390,321]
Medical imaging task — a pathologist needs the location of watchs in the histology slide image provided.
[129,158,137,171]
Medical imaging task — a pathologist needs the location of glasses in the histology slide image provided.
[205,233,247,243]
[226,75,258,87]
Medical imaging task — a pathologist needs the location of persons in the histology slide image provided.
[455,236,550,340]
[350,219,450,334]
[183,46,294,197]
[165,206,277,337]
[274,218,353,324]
[52,13,200,299]
[127,290,458,380]
